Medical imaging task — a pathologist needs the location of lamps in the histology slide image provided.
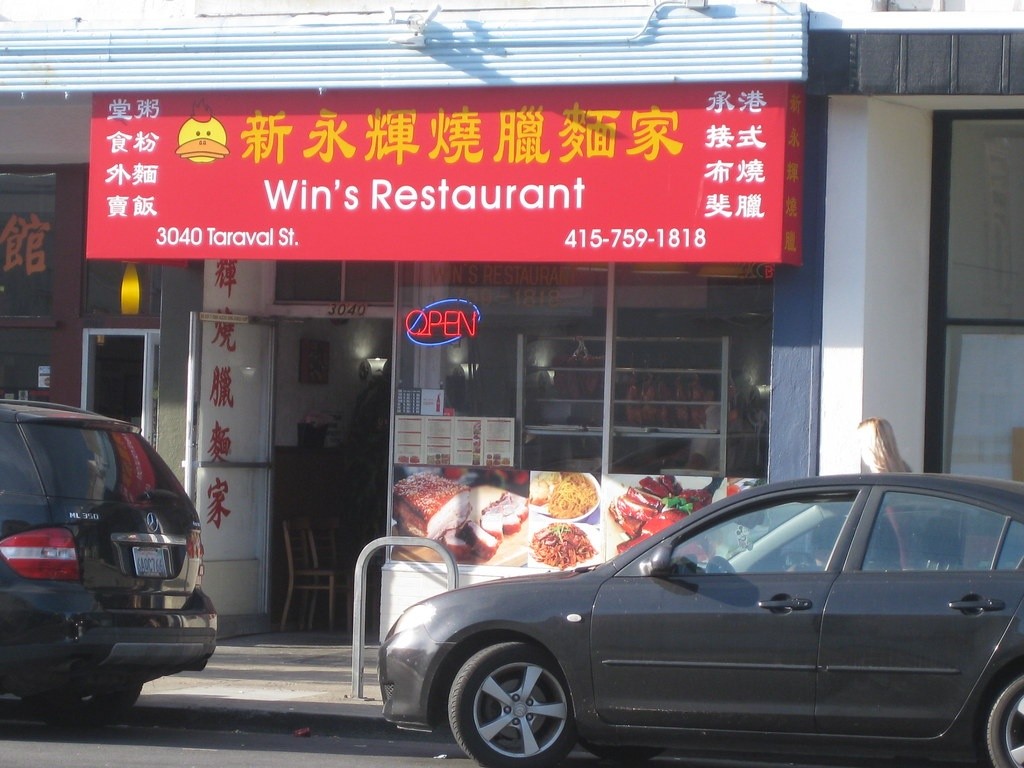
[367,356,388,378]
[460,360,479,381]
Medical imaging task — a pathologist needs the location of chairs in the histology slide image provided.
[276,517,353,634]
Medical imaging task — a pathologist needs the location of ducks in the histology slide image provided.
[550,355,741,429]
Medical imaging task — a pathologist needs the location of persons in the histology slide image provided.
[809,515,844,568]
[851,416,923,571]
[682,361,756,478]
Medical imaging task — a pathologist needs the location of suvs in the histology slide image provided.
[0,396,216,715]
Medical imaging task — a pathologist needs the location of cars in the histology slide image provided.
[377,472,1024,766]
